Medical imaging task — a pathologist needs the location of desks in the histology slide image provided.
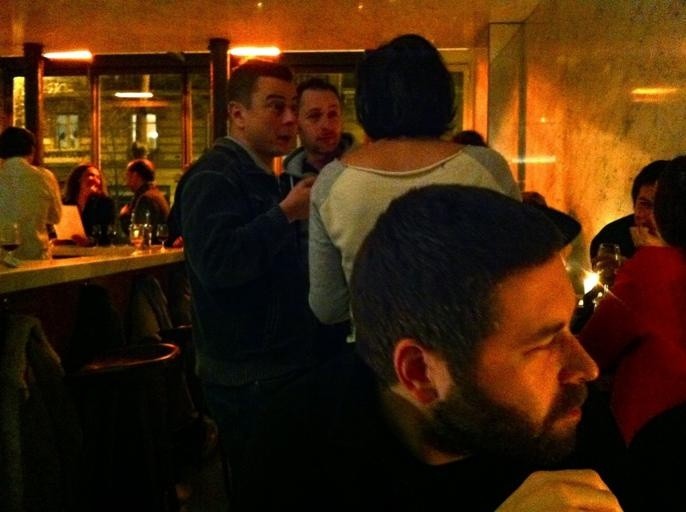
[0,241,183,294]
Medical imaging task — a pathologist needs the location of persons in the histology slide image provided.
[577,156,686,512]
[0,125,62,260]
[307,33,527,325]
[180,61,316,511]
[52,165,116,247]
[118,159,171,244]
[165,147,210,247]
[350,182,629,512]
[126,141,154,167]
[280,77,363,202]
[590,160,667,285]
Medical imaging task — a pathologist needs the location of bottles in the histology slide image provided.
[128,212,137,246]
[92,225,102,248]
[144,214,152,247]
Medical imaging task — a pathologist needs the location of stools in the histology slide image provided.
[80,341,182,512]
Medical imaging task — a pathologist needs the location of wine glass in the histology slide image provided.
[592,243,622,304]
[129,224,143,255]
[0,225,25,267]
[157,224,170,251]
[108,225,118,246]
